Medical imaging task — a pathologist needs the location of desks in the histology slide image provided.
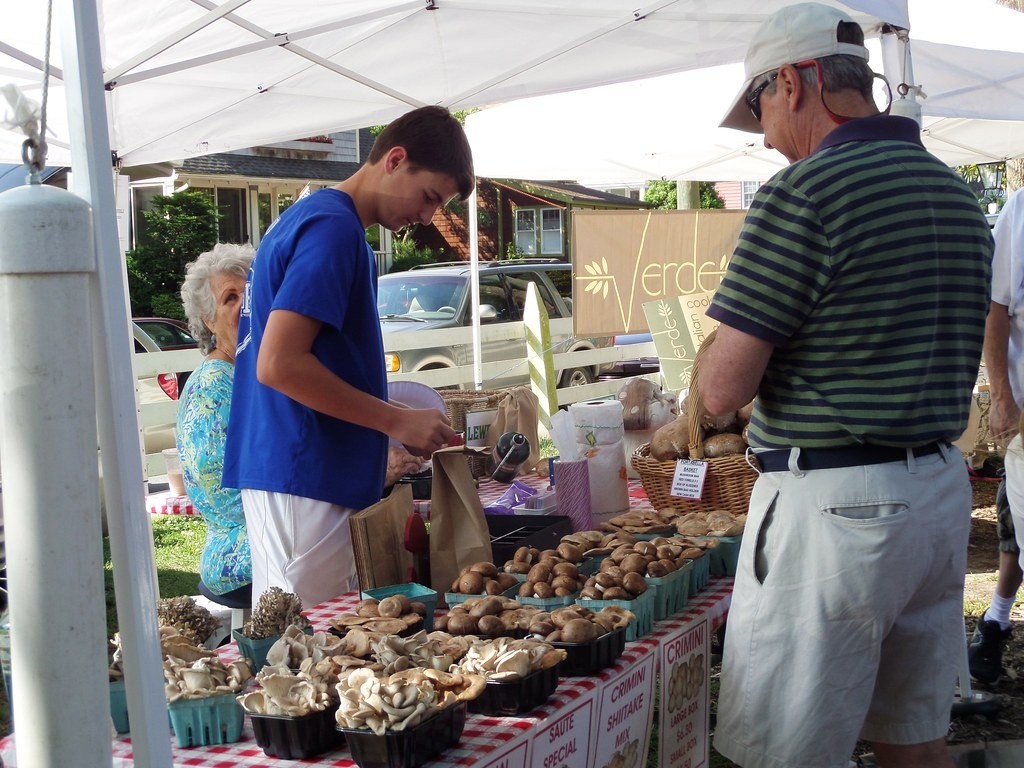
[0,471,733,768]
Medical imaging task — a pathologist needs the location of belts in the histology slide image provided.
[756,438,952,473]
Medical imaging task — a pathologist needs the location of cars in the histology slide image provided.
[131,315,213,453]
[600,333,660,380]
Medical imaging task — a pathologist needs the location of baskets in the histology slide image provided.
[436,388,512,438]
[629,329,760,516]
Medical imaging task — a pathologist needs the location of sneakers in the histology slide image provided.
[968,607,1013,684]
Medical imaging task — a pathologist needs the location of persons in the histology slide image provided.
[175,243,430,596]
[968,186,1024,683]
[697,3,997,768]
[221,106,455,632]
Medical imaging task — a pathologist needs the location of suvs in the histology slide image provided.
[377,255,617,419]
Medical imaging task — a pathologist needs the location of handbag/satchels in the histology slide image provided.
[430,443,493,610]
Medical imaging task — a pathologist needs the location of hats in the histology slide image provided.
[719,2,871,134]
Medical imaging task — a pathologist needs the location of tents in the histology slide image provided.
[0,0,1024,768]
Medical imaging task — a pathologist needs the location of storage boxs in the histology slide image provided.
[444,579,522,611]
[633,529,677,542]
[643,559,694,620]
[510,503,556,515]
[514,590,582,612]
[327,620,424,640]
[449,628,529,639]
[575,584,658,641]
[677,548,713,594]
[550,628,627,672]
[167,691,245,748]
[232,626,313,675]
[110,681,174,733]
[467,663,560,718]
[444,699,469,749]
[576,555,595,576]
[673,532,743,577]
[591,555,608,570]
[510,573,529,582]
[361,581,438,633]
[246,700,347,760]
[335,712,443,768]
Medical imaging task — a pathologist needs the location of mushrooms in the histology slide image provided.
[108,505,747,736]
[647,393,756,461]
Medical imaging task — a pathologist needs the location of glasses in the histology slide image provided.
[747,58,816,123]
